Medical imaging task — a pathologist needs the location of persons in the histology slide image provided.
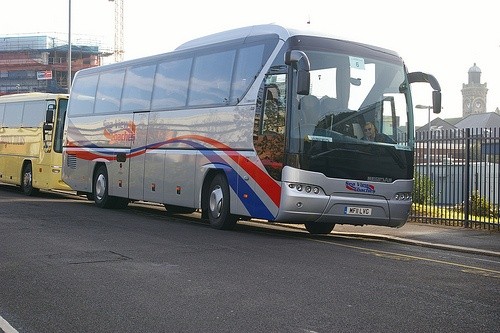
[360,121,397,143]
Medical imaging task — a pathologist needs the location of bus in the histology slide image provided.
[61,24,442,235]
[0,93,92,199]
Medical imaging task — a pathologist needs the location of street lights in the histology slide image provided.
[414,103,444,207]
